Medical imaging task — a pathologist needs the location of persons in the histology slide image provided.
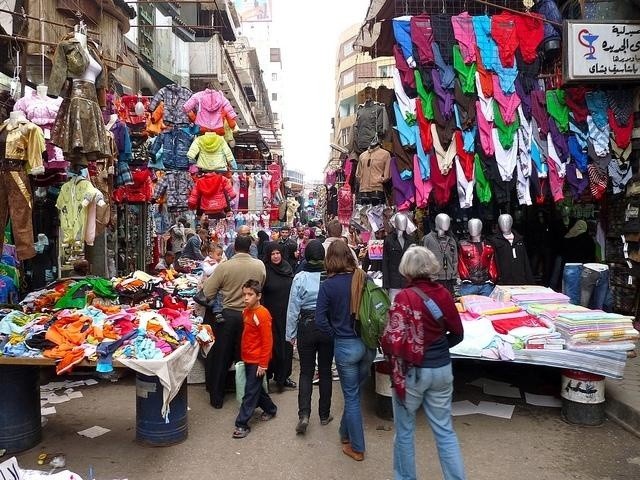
[382,214,418,289]
[562,262,583,306]
[154,217,392,461]
[491,213,535,285]
[421,213,458,292]
[68,259,90,277]
[381,243,466,480]
[580,262,610,310]
[458,217,498,297]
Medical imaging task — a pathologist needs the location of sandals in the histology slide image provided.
[261,412,276,420]
[233,427,249,437]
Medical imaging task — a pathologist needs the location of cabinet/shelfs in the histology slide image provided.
[605,187,640,321]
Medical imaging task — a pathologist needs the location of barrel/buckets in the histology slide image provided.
[0,364,42,453]
[561,370,605,425]
[376,365,393,418]
[135,372,188,446]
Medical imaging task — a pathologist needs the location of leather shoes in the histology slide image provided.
[342,437,349,443]
[343,444,364,461]
[286,378,297,388]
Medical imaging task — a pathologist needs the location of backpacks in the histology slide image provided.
[360,268,391,348]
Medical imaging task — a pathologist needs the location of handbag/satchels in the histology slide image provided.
[235,361,269,401]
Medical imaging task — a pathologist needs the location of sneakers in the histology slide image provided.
[312,371,319,383]
[296,415,308,432]
[216,315,225,323]
[321,415,333,424]
